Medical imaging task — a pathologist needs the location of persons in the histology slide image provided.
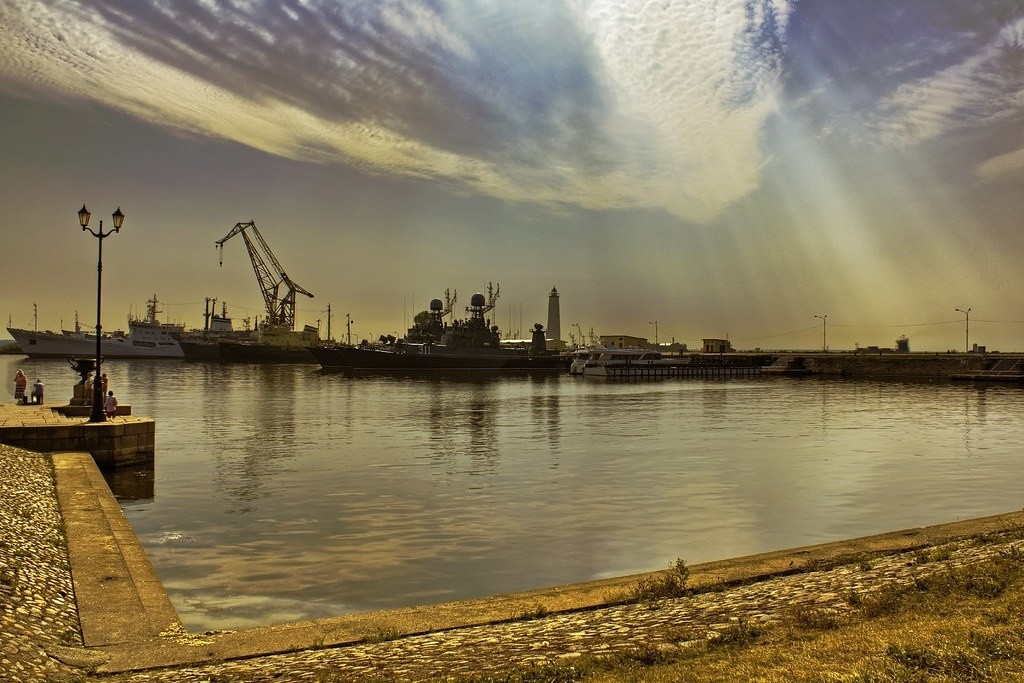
[30,380,44,405]
[14,369,27,405]
[83,372,118,419]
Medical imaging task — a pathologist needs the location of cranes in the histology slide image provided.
[214,219,315,332]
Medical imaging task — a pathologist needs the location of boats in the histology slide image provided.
[760,354,826,377]
[6,291,669,378]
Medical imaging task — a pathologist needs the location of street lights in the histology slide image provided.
[954,307,971,353]
[572,323,581,345]
[814,314,828,353]
[649,321,658,347]
[77,203,126,422]
